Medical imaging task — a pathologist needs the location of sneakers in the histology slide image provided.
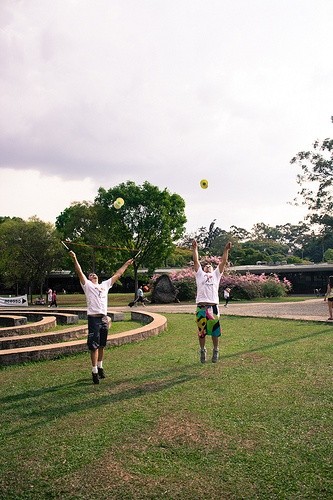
[212,349,218,363]
[201,348,207,363]
[98,368,107,377]
[92,372,99,384]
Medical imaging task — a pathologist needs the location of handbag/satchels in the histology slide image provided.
[101,316,111,329]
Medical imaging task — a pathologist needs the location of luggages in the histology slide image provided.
[128,298,139,307]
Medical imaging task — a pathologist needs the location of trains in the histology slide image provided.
[227,262,333,298]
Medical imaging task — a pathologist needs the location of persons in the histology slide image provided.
[142,282,150,292]
[62,288,66,295]
[135,286,145,307]
[68,250,134,384]
[324,276,333,320]
[45,287,59,307]
[34,297,44,304]
[192,238,240,364]
